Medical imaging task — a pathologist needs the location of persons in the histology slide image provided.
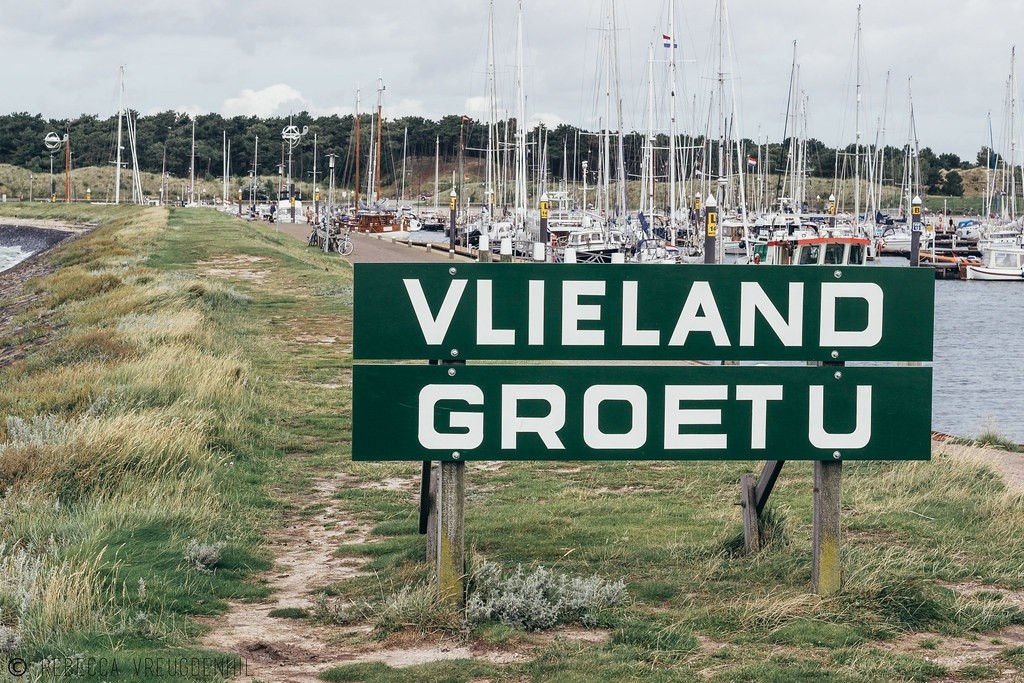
[270,201,277,219]
[587,201,593,209]
[949,217,953,230]
[306,206,311,223]
[439,211,443,218]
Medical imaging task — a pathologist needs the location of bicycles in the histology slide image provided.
[306,214,354,256]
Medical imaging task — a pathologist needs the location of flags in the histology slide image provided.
[662,35,677,48]
[749,157,757,166]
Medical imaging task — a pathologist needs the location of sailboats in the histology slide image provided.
[89,0,1022,283]
